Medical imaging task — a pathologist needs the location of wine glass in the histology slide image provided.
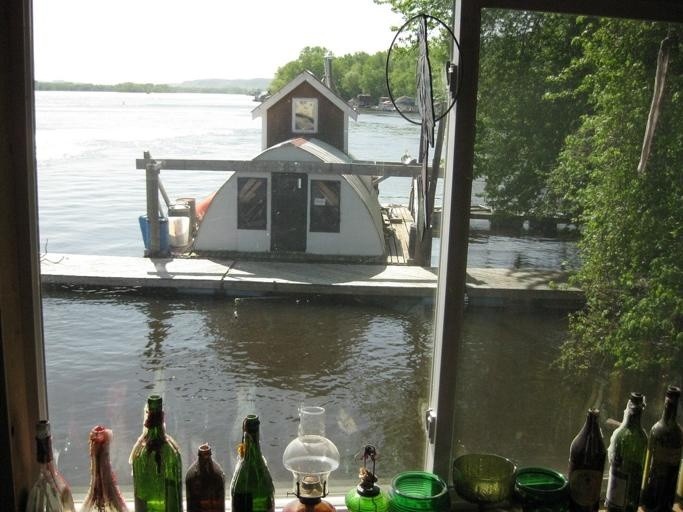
[452,452,515,512]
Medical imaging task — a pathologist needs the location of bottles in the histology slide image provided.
[387,471,448,512]
[24,393,275,511]
[514,465,568,511]
[568,385,683,512]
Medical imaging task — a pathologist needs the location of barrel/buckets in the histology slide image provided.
[139,198,196,253]
